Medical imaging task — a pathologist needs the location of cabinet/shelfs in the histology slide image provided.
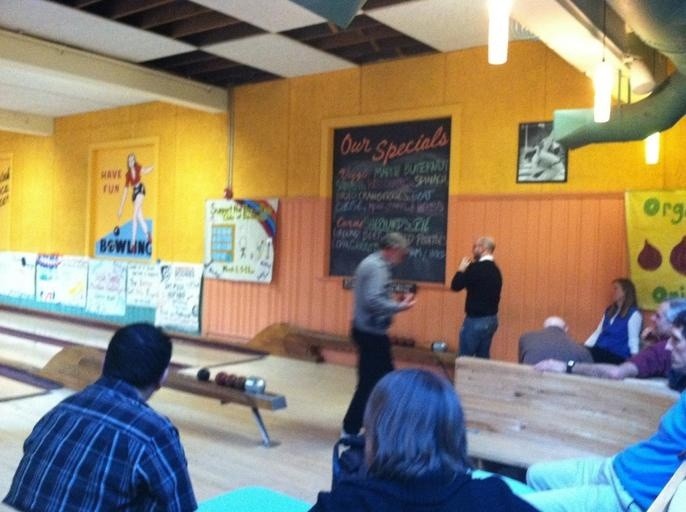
[38,343,287,449]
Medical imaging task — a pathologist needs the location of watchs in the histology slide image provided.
[567,359,577,373]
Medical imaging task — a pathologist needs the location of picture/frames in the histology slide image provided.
[514,120,568,183]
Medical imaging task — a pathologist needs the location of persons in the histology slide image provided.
[583,277,644,366]
[1,318,198,511]
[118,152,155,254]
[518,315,593,366]
[533,298,686,382]
[449,237,502,359]
[337,230,418,440]
[537,134,560,154]
[520,308,686,512]
[524,148,563,180]
[306,365,537,512]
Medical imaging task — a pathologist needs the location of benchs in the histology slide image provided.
[331,352,678,481]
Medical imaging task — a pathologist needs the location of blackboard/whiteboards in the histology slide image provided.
[330,116,452,283]
